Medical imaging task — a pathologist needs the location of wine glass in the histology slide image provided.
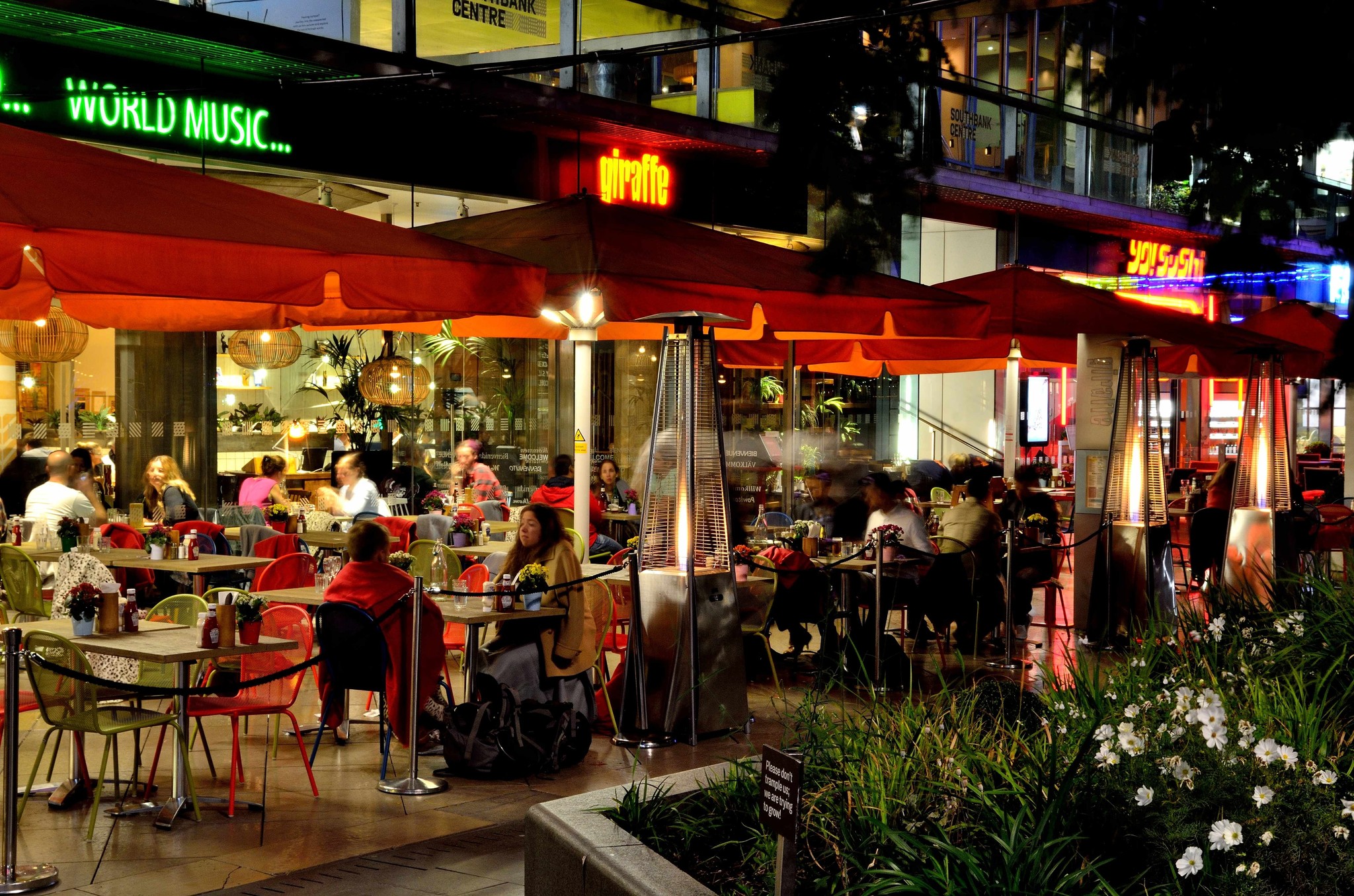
[324,554,342,587]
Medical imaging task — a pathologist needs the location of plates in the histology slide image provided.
[143,521,159,527]
[606,504,628,513]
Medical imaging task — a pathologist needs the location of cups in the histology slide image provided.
[831,536,852,558]
[495,583,516,612]
[165,529,180,559]
[288,514,298,533]
[315,572,329,594]
[76,521,89,545]
[215,604,236,648]
[304,502,315,515]
[35,527,63,552]
[107,503,144,528]
[77,534,91,555]
[452,579,468,609]
[98,536,111,555]
[118,596,128,625]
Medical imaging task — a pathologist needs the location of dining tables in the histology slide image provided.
[0,480,1063,707]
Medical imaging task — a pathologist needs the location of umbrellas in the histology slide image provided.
[382,186,991,565]
[2,117,545,341]
[1220,300,1351,475]
[719,263,1316,477]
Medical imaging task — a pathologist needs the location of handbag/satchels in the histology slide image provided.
[445,685,593,776]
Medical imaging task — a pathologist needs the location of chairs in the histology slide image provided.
[0,662,92,793]
[129,593,216,781]
[583,575,621,737]
[1165,448,1354,633]
[305,601,452,781]
[15,629,202,843]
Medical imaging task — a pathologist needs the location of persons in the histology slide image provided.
[843,475,931,649]
[650,445,676,512]
[533,454,623,564]
[1,433,51,516]
[949,453,995,481]
[0,497,9,543]
[590,459,641,543]
[909,459,949,493]
[997,467,1059,644]
[319,453,378,533]
[939,478,1006,652]
[63,449,109,511]
[239,455,289,528]
[383,443,447,518]
[782,471,846,659]
[1206,462,1261,507]
[320,520,450,750]
[479,503,594,742]
[135,457,204,526]
[24,453,111,579]
[450,440,511,532]
[77,442,106,494]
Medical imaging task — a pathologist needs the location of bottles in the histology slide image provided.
[853,530,876,560]
[779,525,796,554]
[753,504,768,554]
[472,522,491,547]
[611,485,619,513]
[171,529,198,560]
[196,611,208,648]
[279,476,289,501]
[452,481,460,505]
[597,481,607,512]
[448,508,457,520]
[482,580,495,612]
[499,574,514,613]
[124,588,140,632]
[11,516,22,546]
[431,536,448,602]
[297,507,307,534]
[93,528,102,550]
[203,603,220,649]
[818,523,829,557]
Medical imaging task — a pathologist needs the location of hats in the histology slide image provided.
[812,471,834,483]
[857,472,891,493]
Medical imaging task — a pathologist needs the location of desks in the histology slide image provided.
[248,584,388,737]
[66,627,298,817]
[0,618,188,802]
[143,604,320,816]
